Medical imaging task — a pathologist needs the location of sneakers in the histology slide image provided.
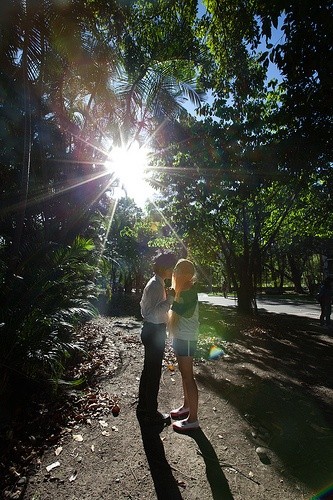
[173,419,198,430]
[170,406,191,416]
[136,408,170,423]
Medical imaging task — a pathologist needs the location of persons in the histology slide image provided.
[137,251,195,420]
[166,259,200,432]
